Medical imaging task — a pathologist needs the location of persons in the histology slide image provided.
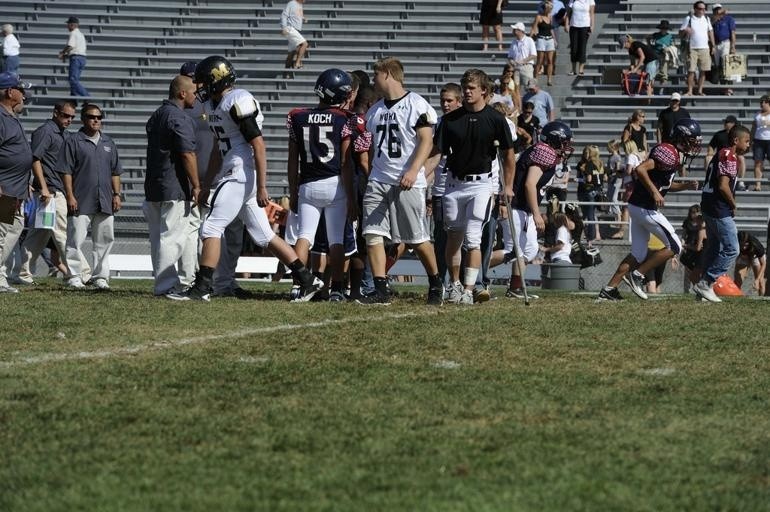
[689,124,750,302]
[494,64,522,129]
[348,70,373,92]
[516,101,542,161]
[577,146,588,219]
[58,16,91,96]
[545,151,570,212]
[565,1,595,76]
[424,69,516,305]
[508,22,536,93]
[488,121,575,300]
[491,76,518,115]
[55,103,122,290]
[2,23,23,100]
[749,94,770,188]
[280,0,309,71]
[178,60,248,293]
[480,0,507,51]
[562,202,583,264]
[551,212,574,265]
[302,71,370,302]
[650,19,677,95]
[682,0,716,96]
[705,115,748,190]
[606,138,623,221]
[585,145,602,239]
[472,78,518,300]
[427,82,465,303]
[529,2,556,86]
[534,0,570,75]
[619,34,660,103]
[0,72,32,292]
[613,139,644,237]
[709,3,738,95]
[350,85,410,301]
[352,56,446,305]
[164,56,327,303]
[488,102,519,160]
[595,117,704,301]
[734,231,767,297]
[658,93,691,147]
[682,214,708,294]
[264,185,291,294]
[639,231,679,294]
[10,100,78,281]
[520,78,555,145]
[145,73,202,303]
[287,68,372,303]
[682,204,704,295]
[623,110,649,151]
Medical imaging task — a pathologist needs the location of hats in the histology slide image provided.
[180,60,197,76]
[23,90,32,99]
[618,36,628,48]
[510,21,525,32]
[711,3,721,8]
[722,115,737,123]
[655,20,672,31]
[1,70,32,89]
[64,16,79,24]
[758,95,768,102]
[526,79,537,90]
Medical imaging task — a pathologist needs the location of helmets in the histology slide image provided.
[193,56,236,103]
[540,121,575,154]
[314,68,352,108]
[673,118,702,169]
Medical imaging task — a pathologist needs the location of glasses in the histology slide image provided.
[524,110,532,115]
[503,82,508,85]
[506,70,514,73]
[640,114,644,117]
[697,7,707,11]
[59,112,76,120]
[85,114,103,120]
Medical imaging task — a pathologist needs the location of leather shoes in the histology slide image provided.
[547,81,553,87]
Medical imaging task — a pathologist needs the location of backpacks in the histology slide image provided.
[579,230,603,269]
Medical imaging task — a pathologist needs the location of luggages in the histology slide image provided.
[670,92,681,101]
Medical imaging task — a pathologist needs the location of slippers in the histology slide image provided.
[683,93,691,97]
[698,92,706,97]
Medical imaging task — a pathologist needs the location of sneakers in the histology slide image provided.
[95,278,110,290]
[692,280,723,303]
[329,292,347,302]
[473,285,489,302]
[446,281,464,303]
[505,288,539,300]
[578,72,584,76]
[689,287,707,302]
[69,276,86,290]
[568,71,575,76]
[428,279,445,308]
[622,270,648,300]
[598,286,628,302]
[461,289,474,305]
[355,294,392,306]
[288,277,324,303]
[166,283,211,302]
[0,285,19,293]
[290,284,301,299]
